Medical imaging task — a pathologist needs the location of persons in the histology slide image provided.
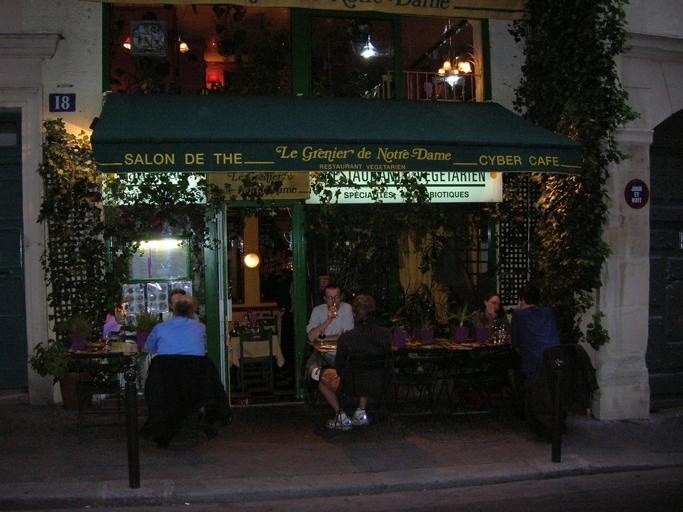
[468,284,560,411]
[306,285,391,430]
[145,288,207,356]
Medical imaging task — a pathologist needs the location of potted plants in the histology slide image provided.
[446,303,474,343]
[64,315,92,351]
[392,320,408,348]
[415,316,434,344]
[136,312,160,349]
[28,338,95,410]
[473,313,493,342]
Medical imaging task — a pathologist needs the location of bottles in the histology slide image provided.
[231,312,263,343]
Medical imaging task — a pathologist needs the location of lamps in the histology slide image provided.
[438,18,472,88]
[360,34,378,59]
[178,36,189,54]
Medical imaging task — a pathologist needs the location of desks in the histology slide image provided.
[69,343,143,375]
[231,328,285,369]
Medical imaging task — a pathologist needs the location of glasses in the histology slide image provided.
[328,295,340,300]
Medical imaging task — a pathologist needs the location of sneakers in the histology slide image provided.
[325,408,369,430]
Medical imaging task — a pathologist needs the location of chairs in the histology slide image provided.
[308,343,580,446]
[257,315,278,336]
[236,332,276,394]
[146,354,214,450]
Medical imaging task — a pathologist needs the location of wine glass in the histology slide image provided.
[318,327,327,346]
